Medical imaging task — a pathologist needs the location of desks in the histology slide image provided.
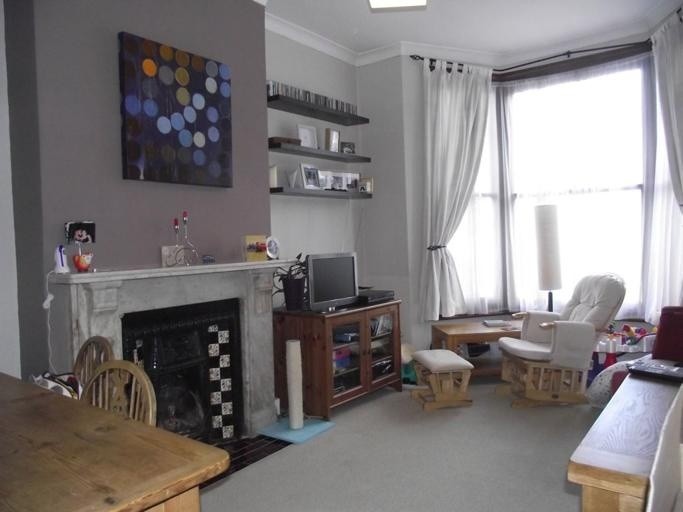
[1,371,229,512]
[431,319,522,355]
[567,372,683,512]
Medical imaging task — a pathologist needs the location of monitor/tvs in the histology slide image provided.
[305,251,360,312]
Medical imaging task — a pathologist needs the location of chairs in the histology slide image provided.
[496,273,626,407]
[73,334,114,389]
[79,361,157,427]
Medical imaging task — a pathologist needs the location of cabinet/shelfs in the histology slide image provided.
[268,80,374,200]
[273,298,403,421]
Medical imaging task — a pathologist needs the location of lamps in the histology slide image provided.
[368,0,428,14]
[534,205,563,313]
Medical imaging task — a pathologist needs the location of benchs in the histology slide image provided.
[410,347,475,412]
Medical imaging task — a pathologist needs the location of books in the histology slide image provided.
[266,80,359,116]
